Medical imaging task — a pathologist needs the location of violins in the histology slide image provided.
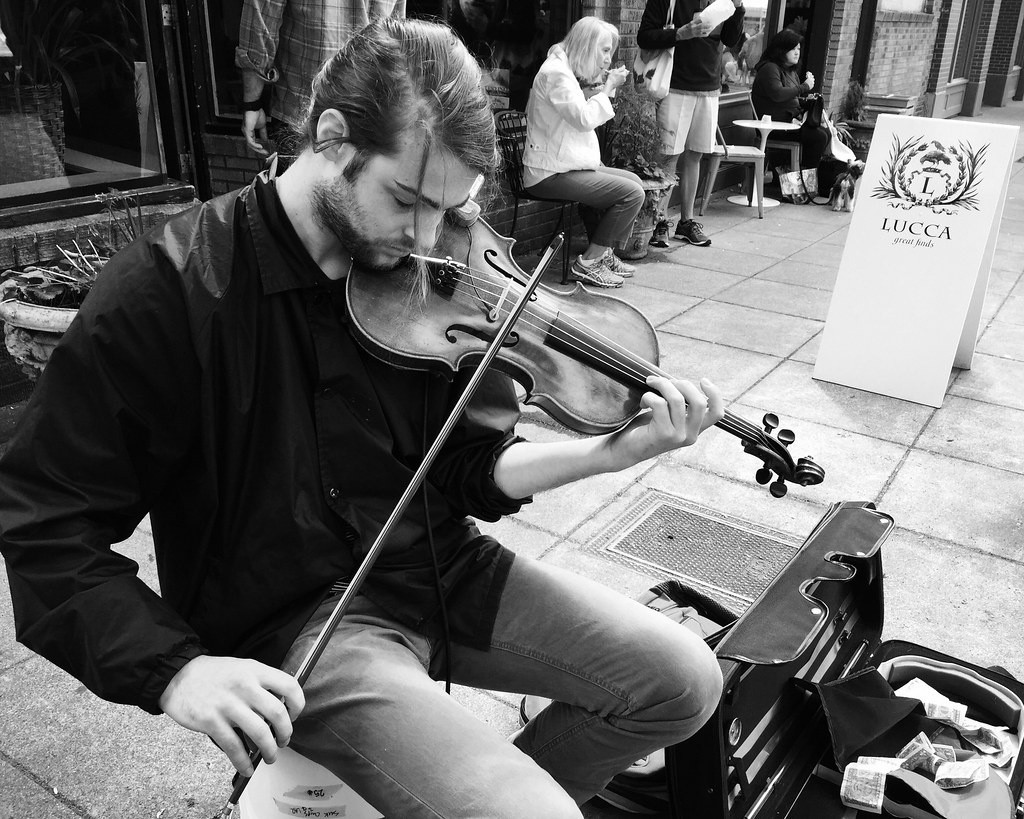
[345,214,825,498]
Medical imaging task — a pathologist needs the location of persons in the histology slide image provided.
[520,16,647,290]
[236,0,410,175]
[637,0,745,247]
[0,19,723,819]
[751,27,873,196]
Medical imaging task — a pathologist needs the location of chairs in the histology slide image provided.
[749,93,803,205]
[495,110,593,285]
[696,124,766,219]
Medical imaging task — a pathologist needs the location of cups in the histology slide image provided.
[693,12,701,21]
[762,115,771,123]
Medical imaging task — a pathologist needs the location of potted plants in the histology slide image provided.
[580,83,680,259]
[0,0,143,185]
[0,187,144,382]
[841,79,877,163]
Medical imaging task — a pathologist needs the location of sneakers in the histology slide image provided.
[649,221,675,247]
[598,247,636,278]
[673,219,712,246]
[571,253,626,289]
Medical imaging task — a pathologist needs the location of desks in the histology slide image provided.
[727,119,801,207]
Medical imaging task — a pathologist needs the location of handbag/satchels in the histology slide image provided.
[796,91,824,128]
[633,23,676,101]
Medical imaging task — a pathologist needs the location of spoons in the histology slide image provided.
[603,68,630,73]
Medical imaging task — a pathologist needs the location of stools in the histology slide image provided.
[239,746,385,819]
